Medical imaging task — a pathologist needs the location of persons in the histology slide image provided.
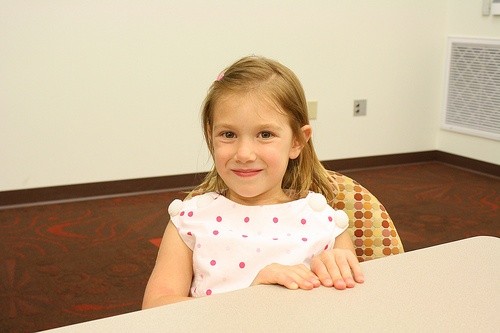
[142,55,364,311]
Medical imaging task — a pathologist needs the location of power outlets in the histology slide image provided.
[307,101,318,120]
[353,99,367,116]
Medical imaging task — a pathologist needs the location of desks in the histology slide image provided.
[31,234,499,333]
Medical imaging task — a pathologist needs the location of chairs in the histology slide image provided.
[181,169,405,264]
